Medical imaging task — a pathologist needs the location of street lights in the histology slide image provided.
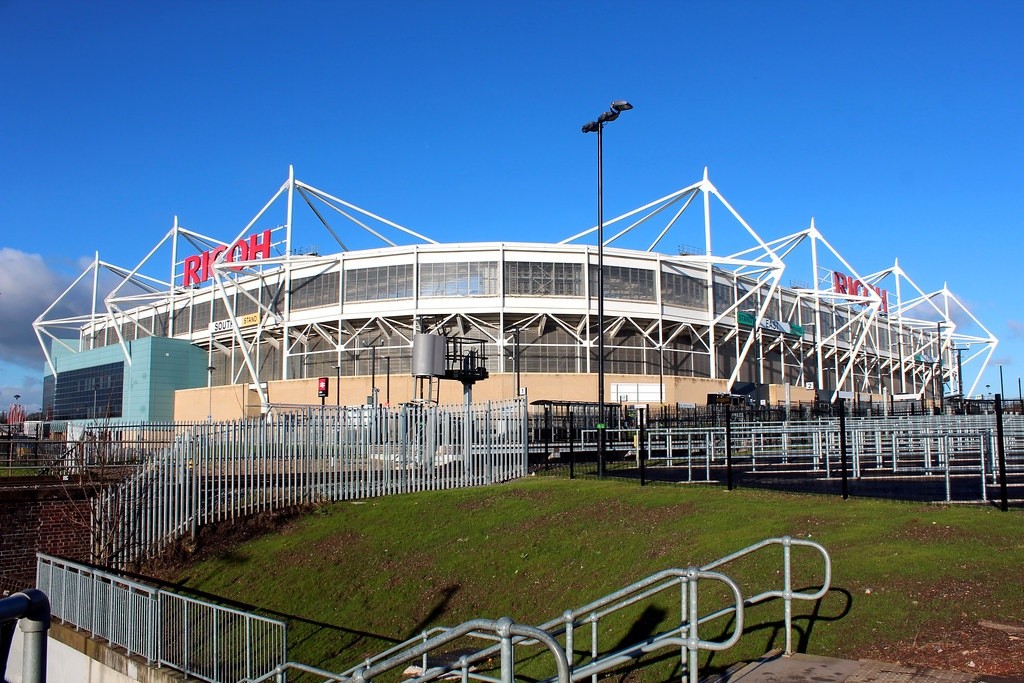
[381,355,389,405]
[331,366,341,441]
[583,100,633,478]
[938,320,946,413]
[207,366,216,422]
